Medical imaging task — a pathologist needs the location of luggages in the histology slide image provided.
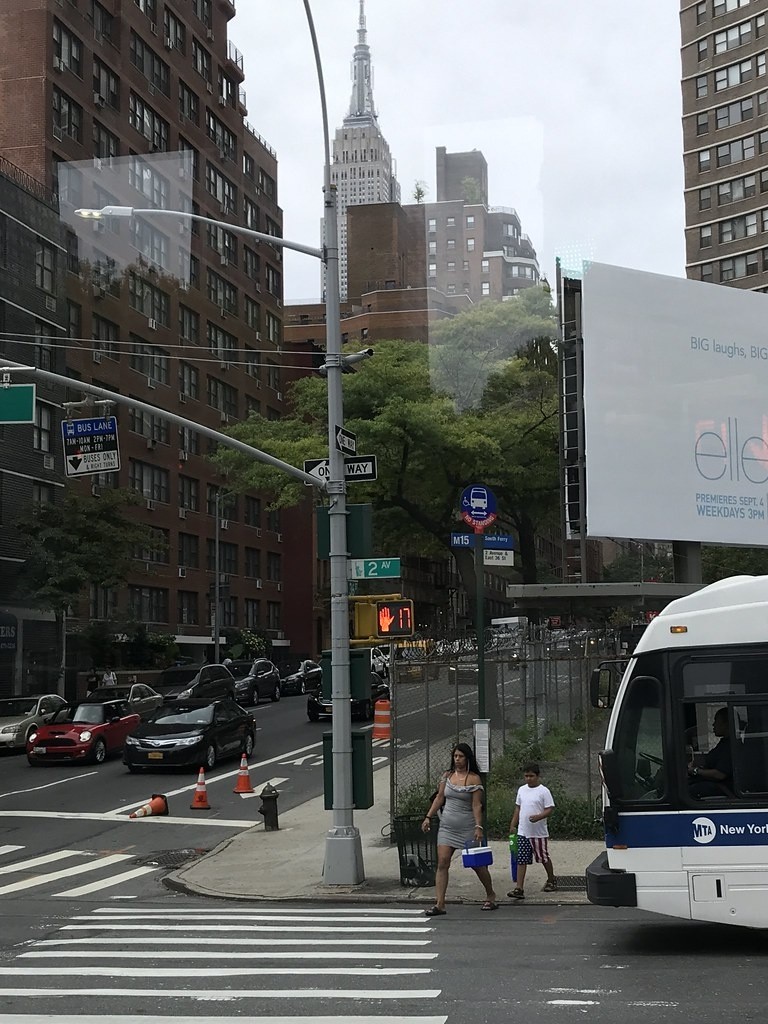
[511,851,518,881]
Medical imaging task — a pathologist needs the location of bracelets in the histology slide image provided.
[426,816,431,820]
[475,825,483,830]
[693,768,698,775]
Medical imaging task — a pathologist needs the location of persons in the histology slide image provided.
[687,707,747,796]
[86,668,100,698]
[422,743,498,915]
[507,764,557,898]
[222,656,232,665]
[102,665,117,687]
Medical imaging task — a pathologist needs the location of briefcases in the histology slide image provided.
[461,838,493,867]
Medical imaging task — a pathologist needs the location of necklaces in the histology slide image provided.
[455,771,465,781]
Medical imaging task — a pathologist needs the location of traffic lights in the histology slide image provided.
[353,602,375,638]
[376,600,415,638]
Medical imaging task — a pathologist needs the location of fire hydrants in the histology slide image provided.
[256,782,280,833]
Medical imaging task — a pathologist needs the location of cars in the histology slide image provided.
[26,698,141,764]
[359,648,389,678]
[86,684,163,721]
[0,693,72,752]
[396,635,604,684]
[306,671,390,720]
[123,696,258,768]
[278,657,323,694]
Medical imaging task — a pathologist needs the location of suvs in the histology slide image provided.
[152,663,237,711]
[228,657,282,706]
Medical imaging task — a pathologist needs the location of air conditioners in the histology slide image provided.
[92,155,101,171]
[94,93,105,108]
[178,450,187,460]
[148,318,157,331]
[220,204,228,215]
[53,55,63,73]
[221,520,229,530]
[92,351,102,364]
[93,221,105,234]
[164,37,172,50]
[278,392,282,401]
[94,287,105,300]
[276,253,282,263]
[179,168,187,180]
[179,507,187,519]
[221,412,229,421]
[149,141,159,153]
[179,568,186,577]
[219,96,226,109]
[206,29,215,41]
[221,256,229,266]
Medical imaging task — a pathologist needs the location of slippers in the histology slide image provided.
[425,906,446,915]
[482,900,499,910]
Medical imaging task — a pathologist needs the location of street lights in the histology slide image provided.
[213,484,250,665]
[73,204,366,886]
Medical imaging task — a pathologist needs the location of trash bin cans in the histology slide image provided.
[390,813,437,888]
[320,731,379,813]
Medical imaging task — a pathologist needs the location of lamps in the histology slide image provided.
[220,151,229,162]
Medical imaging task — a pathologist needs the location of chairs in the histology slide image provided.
[739,723,765,792]
[117,692,126,697]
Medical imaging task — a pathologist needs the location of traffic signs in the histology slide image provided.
[332,424,359,456]
[301,456,379,485]
[60,415,121,477]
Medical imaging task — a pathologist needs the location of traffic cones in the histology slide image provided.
[233,755,256,794]
[128,792,170,819]
[190,767,211,809]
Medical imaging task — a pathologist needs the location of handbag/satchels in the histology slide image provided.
[508,828,518,851]
[429,790,446,820]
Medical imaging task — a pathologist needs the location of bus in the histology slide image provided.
[584,573,767,931]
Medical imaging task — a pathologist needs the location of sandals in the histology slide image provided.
[507,888,525,898]
[541,880,557,892]
